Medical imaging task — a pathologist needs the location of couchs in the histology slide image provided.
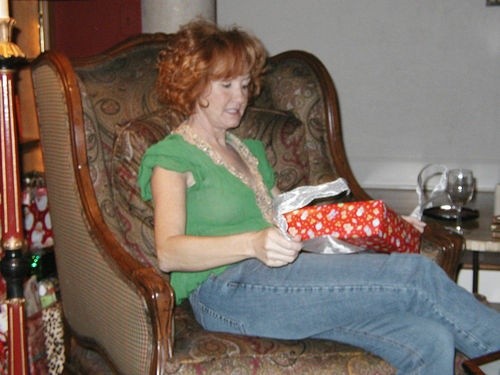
[31,33,491,375]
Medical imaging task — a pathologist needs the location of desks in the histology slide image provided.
[421,210,500,294]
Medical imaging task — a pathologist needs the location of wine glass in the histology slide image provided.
[447,168,474,236]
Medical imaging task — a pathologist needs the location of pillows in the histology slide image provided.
[115,104,310,281]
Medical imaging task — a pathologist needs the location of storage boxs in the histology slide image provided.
[284,200,422,254]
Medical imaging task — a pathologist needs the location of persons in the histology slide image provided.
[138,16,500,375]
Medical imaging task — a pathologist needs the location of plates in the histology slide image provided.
[423,204,480,224]
